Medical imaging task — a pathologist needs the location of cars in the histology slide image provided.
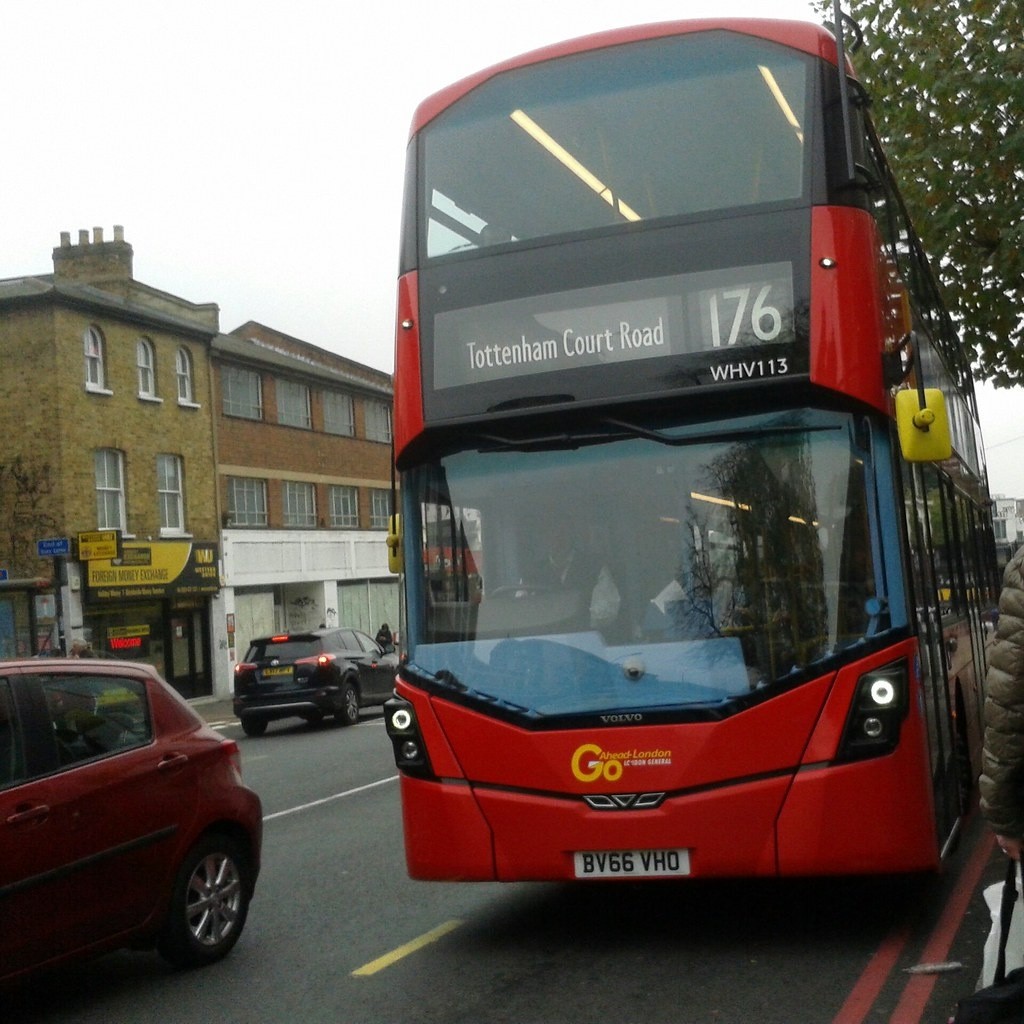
[0,657,262,1006]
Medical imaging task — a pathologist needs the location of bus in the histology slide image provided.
[384,2,1007,884]
[423,515,488,592]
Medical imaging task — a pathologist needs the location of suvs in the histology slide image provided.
[232,627,398,735]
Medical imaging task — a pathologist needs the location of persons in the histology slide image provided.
[375,622,394,655]
[514,538,621,630]
[663,573,777,640]
[67,638,100,659]
[978,546,1024,893]
